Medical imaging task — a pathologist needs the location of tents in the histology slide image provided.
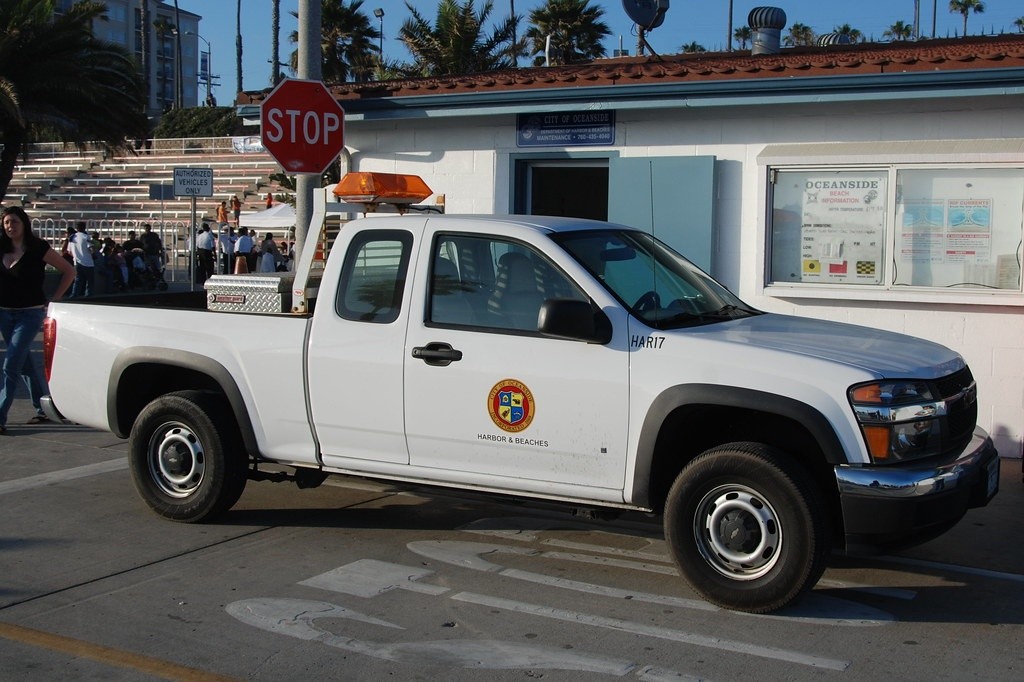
[238,182,341,258]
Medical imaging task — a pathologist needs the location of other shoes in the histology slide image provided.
[0,425,7,432]
[27,414,46,424]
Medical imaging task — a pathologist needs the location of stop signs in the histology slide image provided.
[263,79,345,178]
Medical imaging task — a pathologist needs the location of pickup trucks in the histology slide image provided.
[39,172,1000,615]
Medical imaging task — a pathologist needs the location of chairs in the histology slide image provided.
[489,251,544,329]
[431,256,475,324]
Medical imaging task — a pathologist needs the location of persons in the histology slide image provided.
[62,193,296,302]
[0,206,78,434]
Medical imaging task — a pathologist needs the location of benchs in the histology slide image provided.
[0,156,280,260]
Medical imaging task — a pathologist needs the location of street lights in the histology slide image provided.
[375,9,388,64]
[171,28,183,113]
[185,27,212,107]
